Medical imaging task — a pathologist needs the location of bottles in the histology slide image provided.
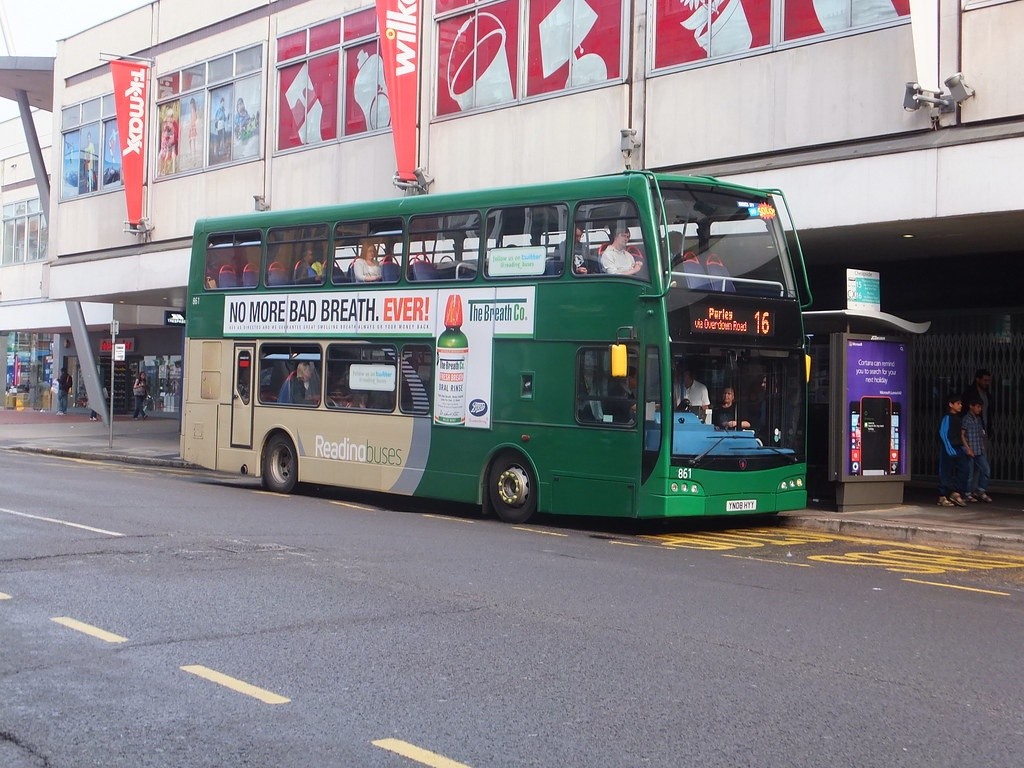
[432,294,469,427]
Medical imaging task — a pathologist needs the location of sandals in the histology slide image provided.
[973,489,993,502]
[937,498,954,506]
[949,493,966,507]
[963,494,978,502]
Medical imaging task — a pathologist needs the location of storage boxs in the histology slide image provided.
[15,393,30,407]
[5,396,16,407]
[43,391,52,410]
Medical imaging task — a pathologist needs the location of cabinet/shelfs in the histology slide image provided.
[96,356,127,414]
[126,356,140,415]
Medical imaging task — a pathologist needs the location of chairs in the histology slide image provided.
[218,264,239,288]
[627,246,647,277]
[268,261,292,285]
[347,262,353,283]
[381,255,400,281]
[294,259,309,285]
[409,253,477,280]
[242,263,260,287]
[276,370,298,404]
[321,258,346,285]
[683,251,713,290]
[351,256,358,283]
[598,242,610,273]
[704,252,736,292]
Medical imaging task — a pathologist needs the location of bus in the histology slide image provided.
[180,168,815,526]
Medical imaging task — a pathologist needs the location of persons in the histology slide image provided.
[599,226,643,275]
[762,374,781,394]
[676,361,710,423]
[160,97,249,173]
[84,132,95,192]
[108,128,118,163]
[662,231,683,268]
[713,387,751,430]
[89,410,97,421]
[608,367,660,412]
[133,372,148,420]
[968,369,992,495]
[555,224,594,274]
[935,396,992,506]
[300,249,325,283]
[56,367,72,416]
[290,362,318,405]
[353,243,383,282]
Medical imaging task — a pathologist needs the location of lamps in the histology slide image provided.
[253,195,270,211]
[944,72,975,105]
[619,129,641,158]
[138,217,155,233]
[413,166,434,191]
[901,80,956,114]
